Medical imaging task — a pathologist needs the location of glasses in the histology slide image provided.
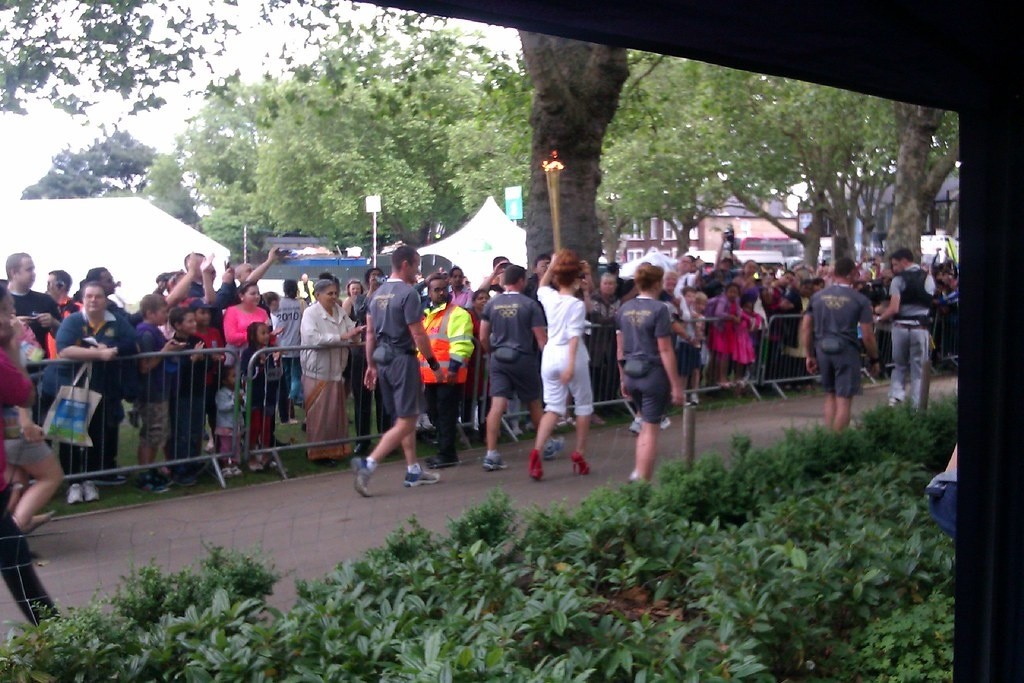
[431,285,448,293]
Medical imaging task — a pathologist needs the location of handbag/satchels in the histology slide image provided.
[494,346,518,363]
[820,337,845,354]
[42,360,102,447]
[624,359,651,379]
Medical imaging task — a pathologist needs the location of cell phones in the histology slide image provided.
[502,263,513,269]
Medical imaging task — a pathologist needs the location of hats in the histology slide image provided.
[188,298,219,312]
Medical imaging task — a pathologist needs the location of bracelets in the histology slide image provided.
[877,316,881,322]
[870,357,880,364]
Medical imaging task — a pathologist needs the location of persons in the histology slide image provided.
[873,247,936,408]
[528,250,595,479]
[802,257,879,433]
[613,262,685,483]
[351,245,442,496]
[415,274,475,468]
[557,232,959,432]
[479,265,564,470]
[0,249,552,534]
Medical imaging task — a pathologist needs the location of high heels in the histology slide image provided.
[528,449,543,480]
[570,450,589,476]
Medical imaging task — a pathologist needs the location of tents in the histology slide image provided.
[0,197,230,303]
[415,196,527,290]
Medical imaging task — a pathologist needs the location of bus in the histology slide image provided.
[740,236,834,264]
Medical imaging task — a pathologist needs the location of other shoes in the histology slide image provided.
[129,408,140,428]
[425,455,461,468]
[354,442,370,454]
[889,397,899,408]
[421,412,605,444]
[95,474,128,486]
[628,470,651,497]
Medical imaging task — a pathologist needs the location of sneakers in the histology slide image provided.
[628,420,641,434]
[83,481,100,501]
[544,437,565,459]
[683,392,699,406]
[414,413,433,431]
[660,417,671,430]
[482,453,508,470]
[404,468,439,487]
[134,417,335,494]
[351,455,373,497]
[66,483,84,504]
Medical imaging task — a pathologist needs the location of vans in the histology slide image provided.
[919,235,959,266]
[683,250,784,276]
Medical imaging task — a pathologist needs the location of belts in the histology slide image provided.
[895,324,927,330]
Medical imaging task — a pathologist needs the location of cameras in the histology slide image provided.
[276,249,290,257]
[375,276,387,284]
[724,230,734,241]
